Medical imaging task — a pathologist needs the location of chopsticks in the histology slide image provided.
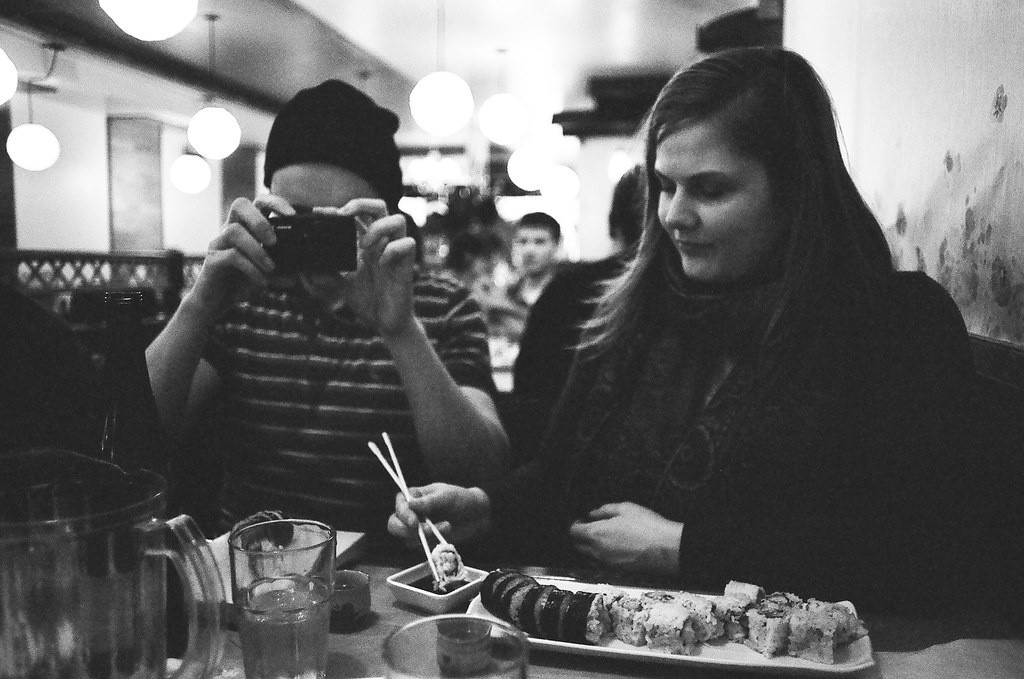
[367,432,466,583]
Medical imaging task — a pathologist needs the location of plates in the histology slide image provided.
[187,530,365,624]
[382,614,530,678]
[466,578,875,677]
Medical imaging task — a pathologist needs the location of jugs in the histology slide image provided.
[1,448,227,679]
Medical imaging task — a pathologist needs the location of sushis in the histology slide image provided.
[232,511,294,577]
[595,580,868,666]
[479,569,605,643]
[432,544,466,591]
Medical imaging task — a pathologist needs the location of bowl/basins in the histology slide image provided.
[386,560,490,613]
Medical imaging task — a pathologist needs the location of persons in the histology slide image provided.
[386,45,1024,614]
[510,165,649,461]
[141,79,513,563]
[443,196,568,342]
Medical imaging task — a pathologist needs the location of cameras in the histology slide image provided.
[267,216,357,272]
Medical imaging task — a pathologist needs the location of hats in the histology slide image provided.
[264,79,404,212]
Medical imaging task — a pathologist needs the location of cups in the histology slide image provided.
[329,570,372,627]
[436,620,492,674]
[227,518,337,679]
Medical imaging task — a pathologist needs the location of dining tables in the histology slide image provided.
[220,537,1023,679]
